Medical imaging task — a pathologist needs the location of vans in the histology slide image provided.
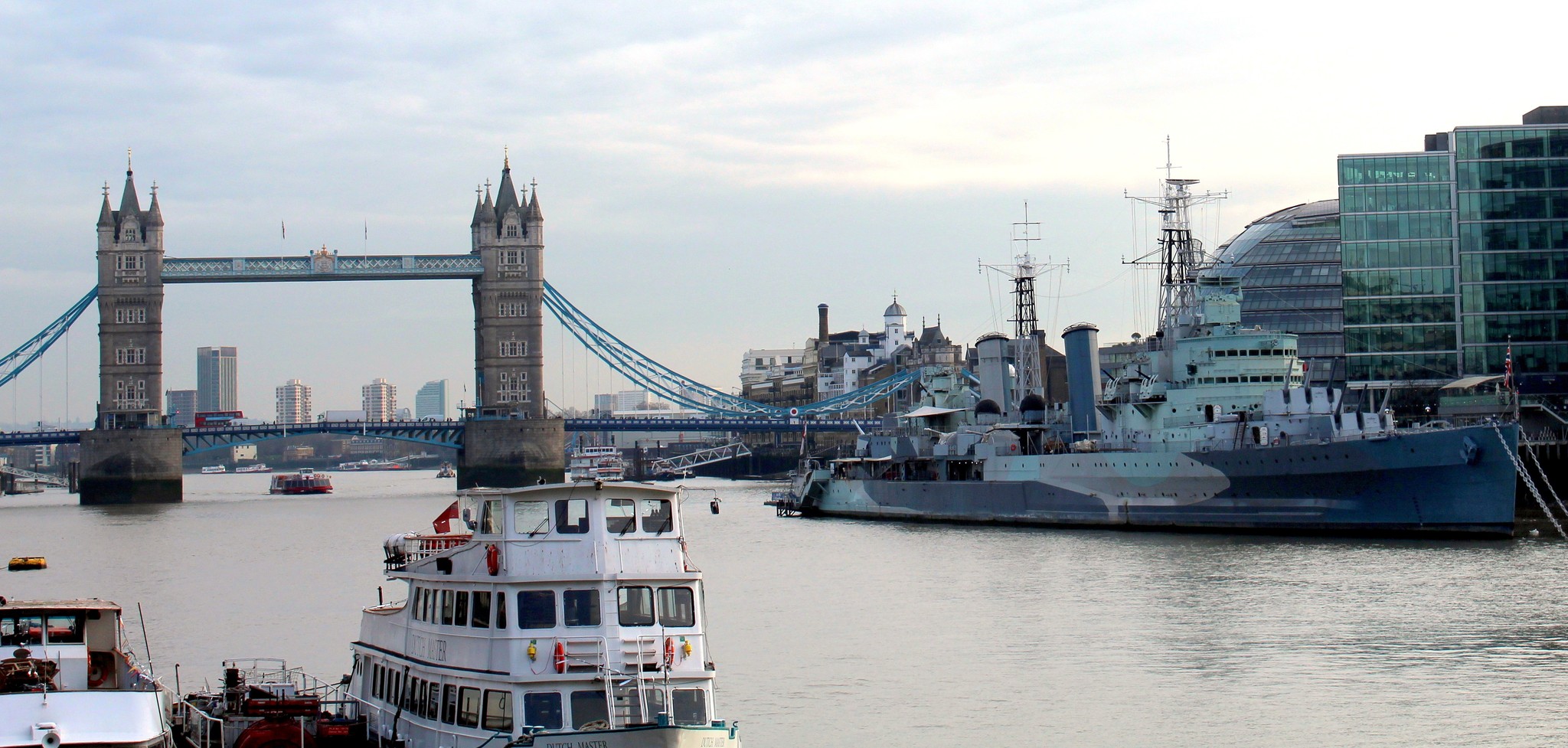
[421,414,444,422]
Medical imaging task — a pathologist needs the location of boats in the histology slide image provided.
[235,463,273,473]
[201,464,227,474]
[759,131,1523,534]
[8,556,47,571]
[164,656,383,748]
[569,431,625,482]
[268,467,333,495]
[339,475,743,748]
[325,459,411,471]
[0,594,175,748]
[436,461,456,478]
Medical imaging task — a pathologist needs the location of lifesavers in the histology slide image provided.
[1011,444,1016,451]
[1273,438,1279,445]
[486,545,498,575]
[87,660,108,687]
[665,637,674,668]
[617,465,620,467]
[554,643,564,673]
[232,719,316,748]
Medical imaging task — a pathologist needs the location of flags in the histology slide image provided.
[433,500,460,534]
[800,421,807,455]
[1504,342,1511,388]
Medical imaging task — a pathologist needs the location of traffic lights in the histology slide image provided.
[38,421,41,427]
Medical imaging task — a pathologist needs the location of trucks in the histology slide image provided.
[317,410,366,423]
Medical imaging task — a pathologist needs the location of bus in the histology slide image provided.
[194,411,244,427]
